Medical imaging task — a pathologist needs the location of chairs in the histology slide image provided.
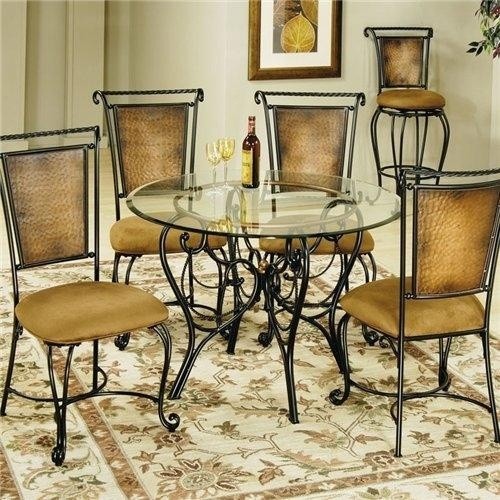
[361,28,450,222]
[253,83,379,354]
[0,125,180,467]
[93,89,232,350]
[329,161,500,455]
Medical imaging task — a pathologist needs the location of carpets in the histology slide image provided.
[0,248,500,500]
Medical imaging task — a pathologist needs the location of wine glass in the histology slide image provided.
[205,142,225,196]
[216,134,235,191]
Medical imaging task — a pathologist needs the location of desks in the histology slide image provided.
[126,170,401,423]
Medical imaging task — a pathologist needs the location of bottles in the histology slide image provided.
[241,113,264,189]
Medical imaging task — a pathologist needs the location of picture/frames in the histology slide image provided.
[248,0,342,81]
